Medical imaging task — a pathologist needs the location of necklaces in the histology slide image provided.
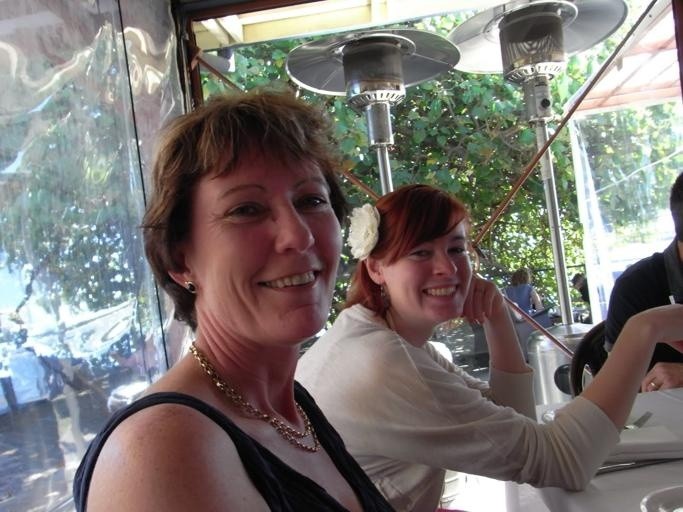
[189,341,320,456]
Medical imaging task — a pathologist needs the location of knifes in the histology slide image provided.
[595,456,675,477]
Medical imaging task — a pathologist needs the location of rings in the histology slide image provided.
[651,379,658,388]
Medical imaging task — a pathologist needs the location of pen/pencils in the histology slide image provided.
[598,461,635,471]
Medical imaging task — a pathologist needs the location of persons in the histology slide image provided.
[6,328,159,474]
[72,93,396,509]
[569,269,588,305]
[292,184,683,512]
[603,172,682,393]
[499,266,541,312]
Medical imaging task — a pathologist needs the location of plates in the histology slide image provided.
[636,483,682,511]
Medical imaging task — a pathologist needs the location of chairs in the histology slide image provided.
[568,319,607,399]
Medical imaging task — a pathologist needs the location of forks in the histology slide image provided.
[624,409,653,433]
